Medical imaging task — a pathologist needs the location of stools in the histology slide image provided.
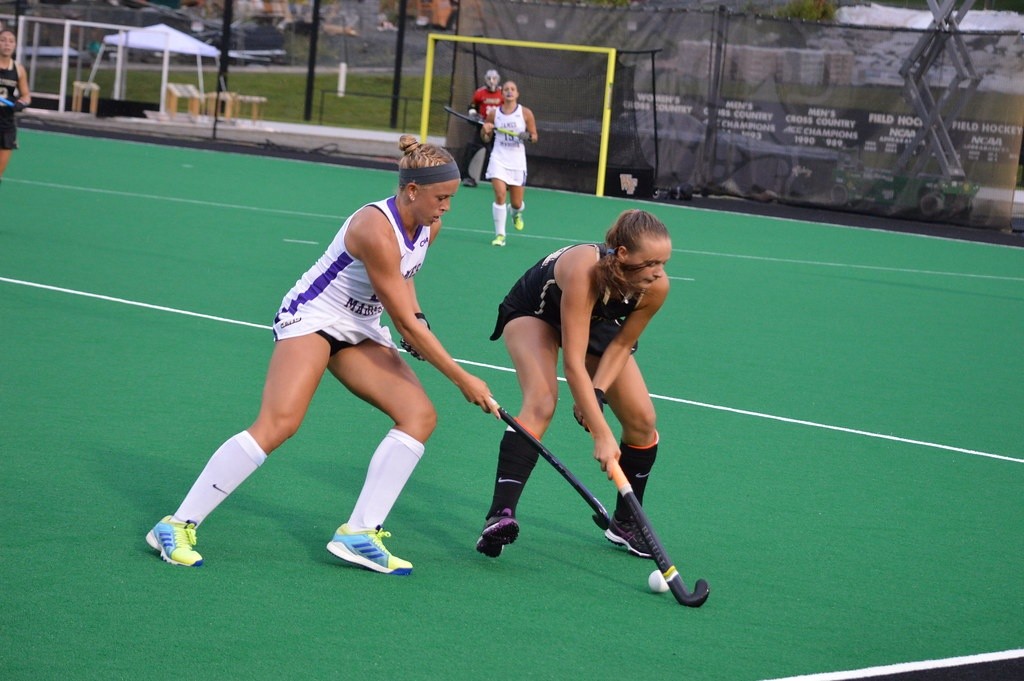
[71,80,100,114]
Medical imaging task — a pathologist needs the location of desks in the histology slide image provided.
[165,83,200,123]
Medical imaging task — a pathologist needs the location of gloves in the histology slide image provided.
[11,101,25,112]
[518,132,530,140]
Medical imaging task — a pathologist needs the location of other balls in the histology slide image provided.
[648,569,670,593]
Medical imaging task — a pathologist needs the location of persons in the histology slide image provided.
[145,135,499,574]
[480,80,538,246]
[0,31,31,176]
[477,209,671,559]
[461,70,504,186]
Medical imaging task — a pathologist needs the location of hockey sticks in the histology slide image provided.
[487,397,710,607]
[443,105,519,137]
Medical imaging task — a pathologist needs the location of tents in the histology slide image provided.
[83,24,221,117]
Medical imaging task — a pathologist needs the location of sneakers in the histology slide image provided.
[146,514,204,568]
[513,212,524,231]
[492,234,506,247]
[604,511,654,558]
[326,523,412,575]
[476,508,518,557]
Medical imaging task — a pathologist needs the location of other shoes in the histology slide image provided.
[464,179,475,187]
